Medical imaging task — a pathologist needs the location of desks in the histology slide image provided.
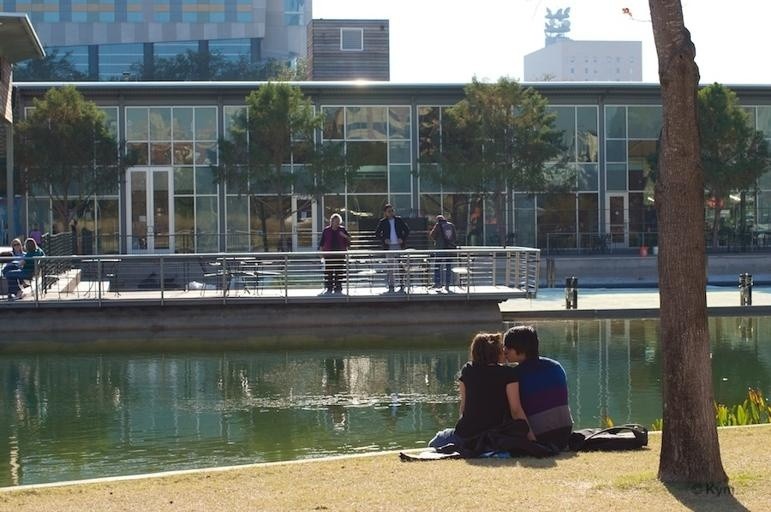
[81,259,121,295]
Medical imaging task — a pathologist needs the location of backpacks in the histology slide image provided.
[571,422,649,450]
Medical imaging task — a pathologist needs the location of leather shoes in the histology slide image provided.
[13,283,31,300]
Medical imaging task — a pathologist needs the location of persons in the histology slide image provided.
[29,224,42,244]
[326,355,350,435]
[425,332,537,449]
[320,213,351,293]
[375,204,409,293]
[504,326,573,451]
[470,205,484,246]
[429,215,458,290]
[4,236,43,298]
[381,352,413,432]
[431,346,457,426]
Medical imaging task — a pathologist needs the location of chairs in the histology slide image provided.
[192,247,477,297]
